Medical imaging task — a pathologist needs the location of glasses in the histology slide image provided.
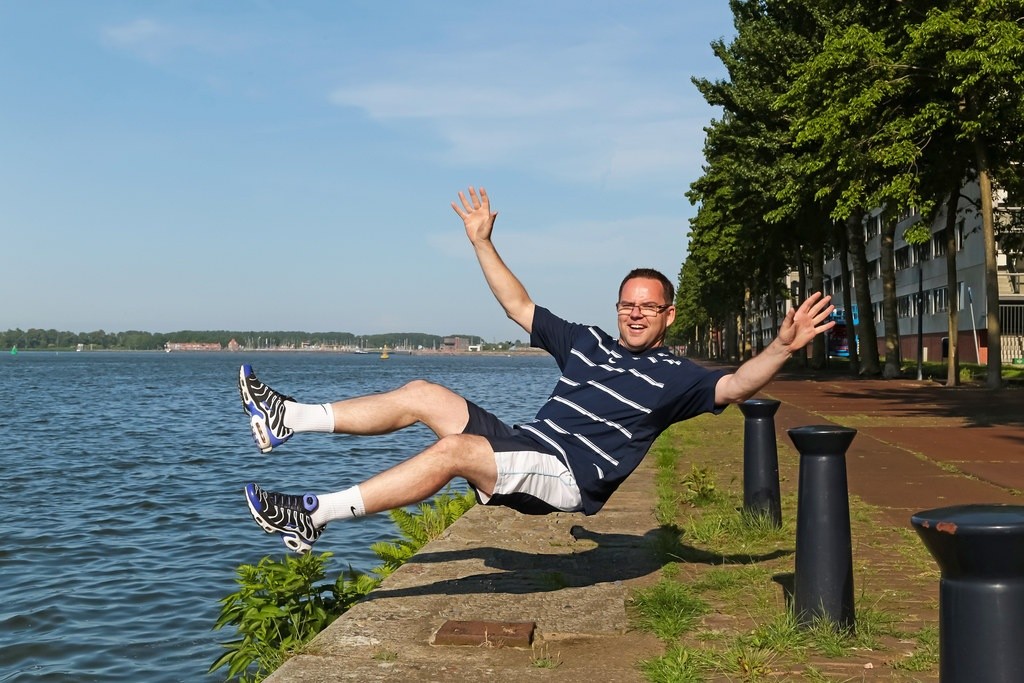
[616,302,669,317]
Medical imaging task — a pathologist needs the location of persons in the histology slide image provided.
[238,184,836,555]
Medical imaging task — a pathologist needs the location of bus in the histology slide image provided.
[823,304,860,359]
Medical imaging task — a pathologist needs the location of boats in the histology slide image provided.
[380,344,389,358]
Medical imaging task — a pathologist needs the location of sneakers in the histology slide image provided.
[244,483,326,555]
[237,364,297,454]
